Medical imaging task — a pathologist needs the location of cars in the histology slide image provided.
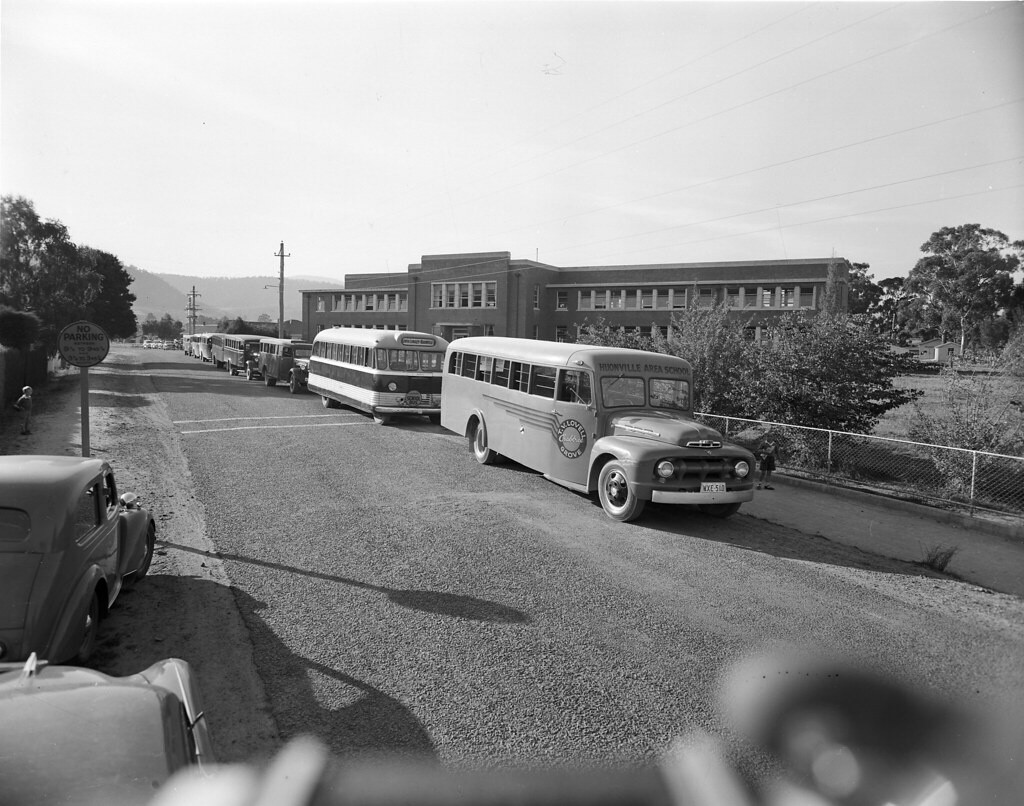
[0,454,156,672]
[0,654,218,806]
[143,339,175,350]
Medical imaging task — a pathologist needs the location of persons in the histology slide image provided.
[13,386,33,436]
[756,436,776,490]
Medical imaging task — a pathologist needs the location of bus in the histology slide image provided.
[257,338,313,394]
[182,332,274,381]
[307,326,449,425]
[440,336,756,524]
